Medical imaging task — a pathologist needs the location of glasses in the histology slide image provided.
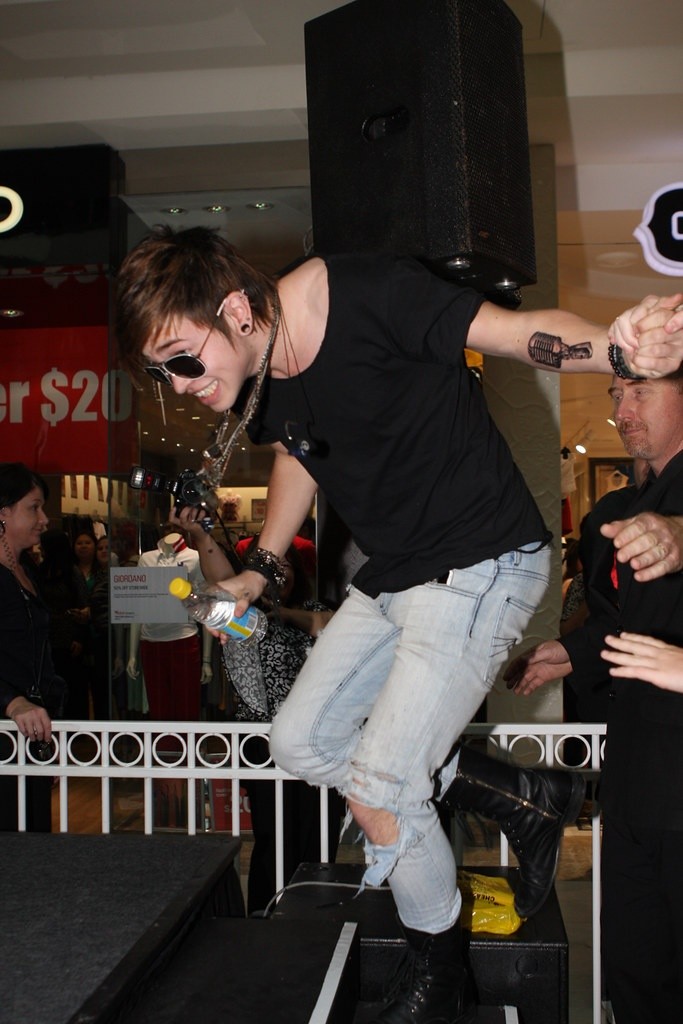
[281,561,292,570]
[142,297,225,386]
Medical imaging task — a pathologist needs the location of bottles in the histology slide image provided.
[168,577,268,646]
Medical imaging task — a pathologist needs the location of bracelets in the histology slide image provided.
[244,546,287,591]
[608,341,647,380]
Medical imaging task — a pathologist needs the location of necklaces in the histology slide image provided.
[196,287,281,498]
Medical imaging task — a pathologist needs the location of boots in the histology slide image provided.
[367,906,478,1024]
[437,745,587,919]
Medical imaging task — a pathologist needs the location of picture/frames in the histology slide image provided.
[588,456,635,511]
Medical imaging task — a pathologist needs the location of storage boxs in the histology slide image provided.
[264,859,570,1024]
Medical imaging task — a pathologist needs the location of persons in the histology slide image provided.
[19,528,122,720]
[126,533,213,721]
[503,358,683,1024]
[0,462,52,832]
[114,222,683,1024]
[168,506,335,722]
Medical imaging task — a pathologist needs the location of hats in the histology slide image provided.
[292,535,316,572]
[238,531,248,538]
